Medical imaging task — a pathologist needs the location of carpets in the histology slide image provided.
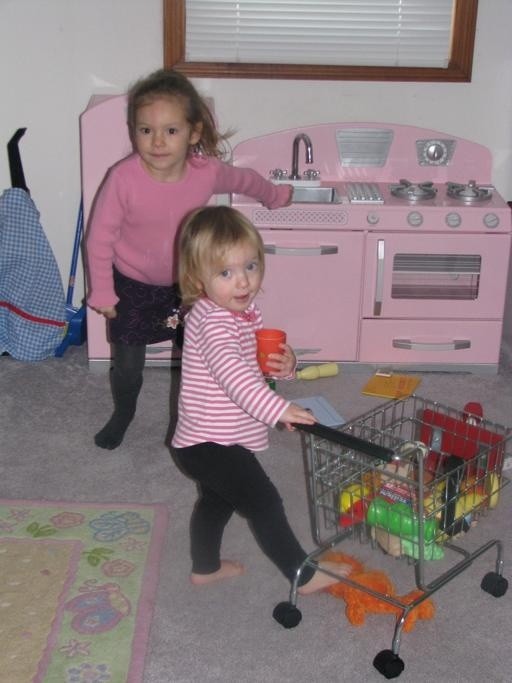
[0,495,171,683]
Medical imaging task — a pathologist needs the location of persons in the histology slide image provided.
[81,68,296,449]
[171,203,352,594]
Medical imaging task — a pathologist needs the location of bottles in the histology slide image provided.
[293,360,343,383]
[363,493,439,543]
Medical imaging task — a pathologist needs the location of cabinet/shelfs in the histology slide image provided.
[253,228,365,366]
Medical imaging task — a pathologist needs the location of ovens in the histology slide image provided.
[362,231,512,319]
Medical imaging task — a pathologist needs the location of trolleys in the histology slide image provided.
[265,390,511,681]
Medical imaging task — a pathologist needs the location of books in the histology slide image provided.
[288,395,346,427]
[361,372,423,400]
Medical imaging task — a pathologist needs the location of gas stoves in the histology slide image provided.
[385,175,502,205]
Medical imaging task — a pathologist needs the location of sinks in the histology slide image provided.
[291,185,343,205]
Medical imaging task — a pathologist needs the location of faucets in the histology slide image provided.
[287,133,313,180]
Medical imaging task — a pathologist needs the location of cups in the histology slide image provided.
[253,328,291,377]
